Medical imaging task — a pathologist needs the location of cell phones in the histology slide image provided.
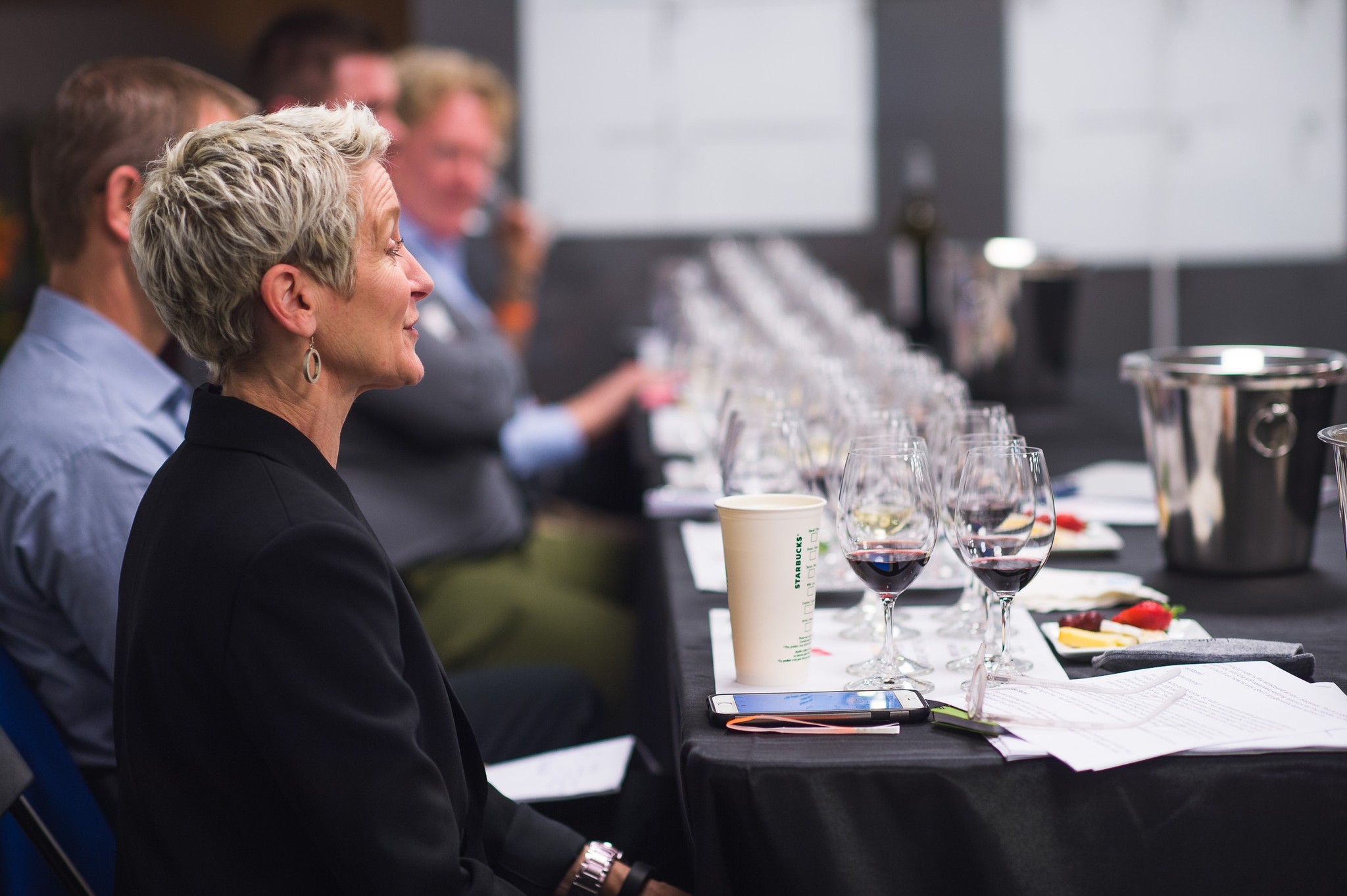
[706,690,930,727]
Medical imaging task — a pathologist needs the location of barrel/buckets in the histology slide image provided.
[1316,423,1347,558]
[953,259,1077,411]
[1118,343,1347,579]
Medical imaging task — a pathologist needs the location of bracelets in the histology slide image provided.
[570,839,625,896]
[619,863,655,896]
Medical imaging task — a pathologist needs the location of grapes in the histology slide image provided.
[1058,610,1101,631]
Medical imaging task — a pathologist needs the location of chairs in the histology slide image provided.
[0,664,122,896]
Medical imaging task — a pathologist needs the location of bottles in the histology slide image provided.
[882,148,954,368]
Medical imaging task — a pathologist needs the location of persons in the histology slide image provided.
[0,56,262,837]
[238,3,654,744]
[119,102,681,896]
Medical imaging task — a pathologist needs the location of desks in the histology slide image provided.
[617,328,1347,896]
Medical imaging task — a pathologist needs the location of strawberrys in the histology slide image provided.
[1110,601,1184,631]
[1054,514,1087,531]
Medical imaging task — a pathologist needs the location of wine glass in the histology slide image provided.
[643,235,1057,701]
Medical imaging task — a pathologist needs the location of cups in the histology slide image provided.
[714,493,831,688]
